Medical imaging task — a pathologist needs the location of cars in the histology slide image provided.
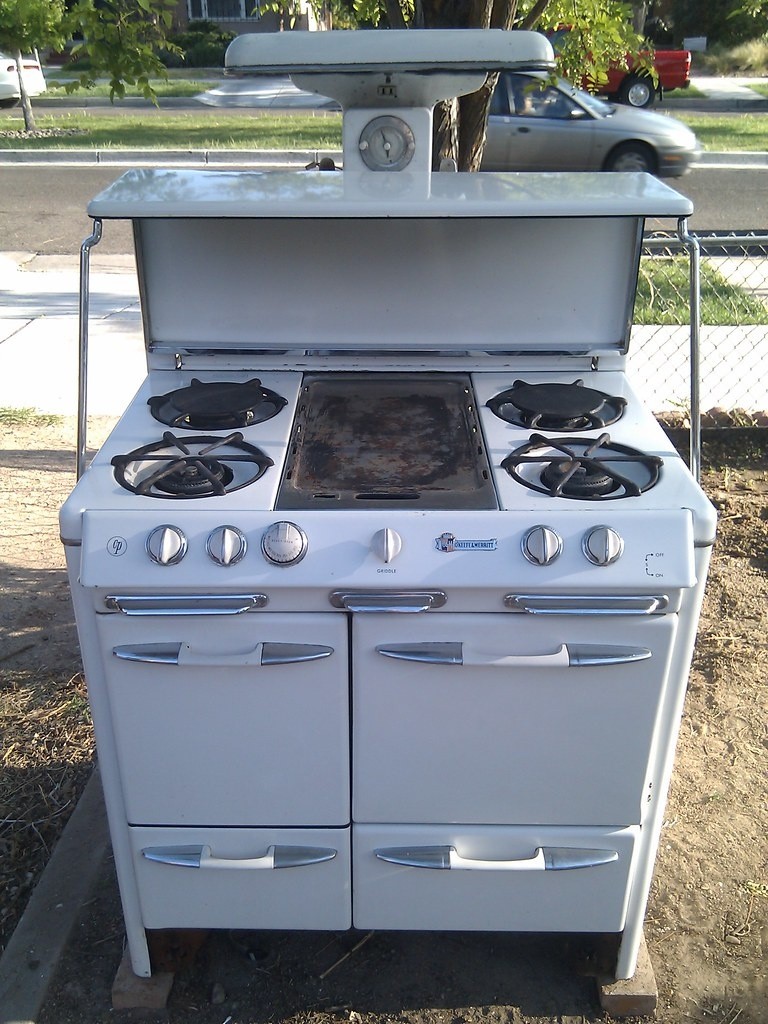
[0,51,46,109]
[480,69,706,181]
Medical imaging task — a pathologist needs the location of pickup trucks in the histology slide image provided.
[543,23,691,110]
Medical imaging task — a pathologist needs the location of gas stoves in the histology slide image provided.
[87,366,692,614]
